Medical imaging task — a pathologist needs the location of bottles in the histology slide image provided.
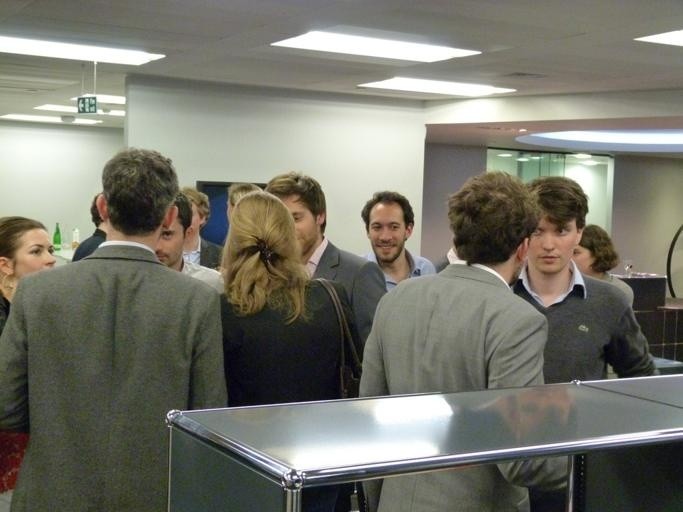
[53,222,61,252]
[71,226,81,250]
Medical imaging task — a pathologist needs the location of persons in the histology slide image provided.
[155,183,260,294]
[1,216,55,512]
[264,172,388,347]
[73,194,106,262]
[0,147,231,512]
[362,191,436,291]
[573,225,634,308]
[219,190,362,512]
[360,171,570,512]
[512,175,660,512]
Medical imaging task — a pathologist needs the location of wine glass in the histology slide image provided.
[622,259,633,278]
